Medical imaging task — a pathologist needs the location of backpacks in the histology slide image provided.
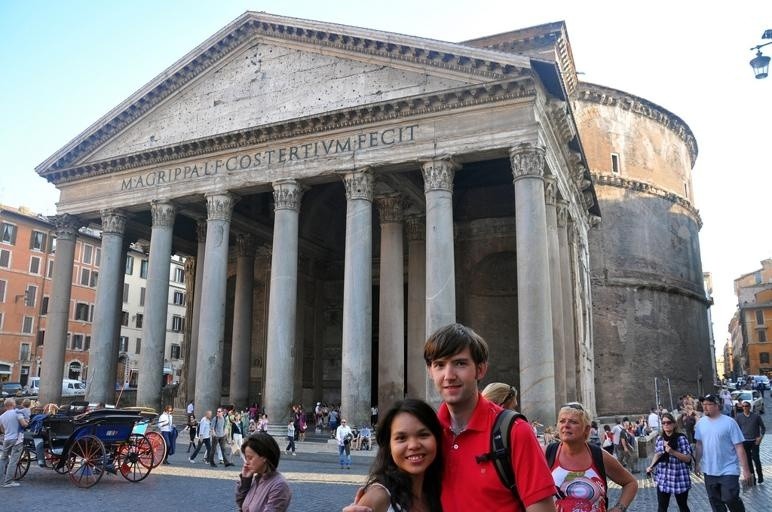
[215,416,229,430]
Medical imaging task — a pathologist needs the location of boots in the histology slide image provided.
[186,443,192,452]
[192,443,196,449]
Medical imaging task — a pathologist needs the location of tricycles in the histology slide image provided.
[5,406,167,488]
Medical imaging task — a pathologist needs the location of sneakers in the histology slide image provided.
[622,464,626,466]
[3,483,21,488]
[293,451,295,456]
[631,468,638,471]
[203,457,208,461]
[210,463,215,466]
[285,450,286,453]
[346,465,350,468]
[188,456,193,459]
[341,465,344,468]
[226,462,231,464]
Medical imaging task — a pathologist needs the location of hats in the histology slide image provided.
[738,397,744,400]
[246,432,282,471]
[316,402,319,405]
[698,394,724,410]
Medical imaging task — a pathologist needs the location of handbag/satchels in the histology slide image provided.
[603,431,611,451]
[331,410,339,421]
[589,435,600,446]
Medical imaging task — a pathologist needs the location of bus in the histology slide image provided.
[129,369,173,388]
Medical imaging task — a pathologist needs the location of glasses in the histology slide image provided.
[497,384,514,408]
[217,411,220,413]
[698,400,714,404]
[341,420,346,422]
[743,404,748,407]
[190,416,192,417]
[167,408,170,410]
[560,402,586,412]
[662,420,676,426]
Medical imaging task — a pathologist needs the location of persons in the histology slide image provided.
[116,381,120,389]
[188,410,212,464]
[313,401,315,418]
[590,421,603,447]
[636,404,647,437]
[351,425,361,451]
[125,381,129,388]
[715,370,759,394]
[249,402,261,419]
[185,413,198,454]
[759,380,766,397]
[323,403,329,426]
[300,403,308,442]
[531,419,558,442]
[359,423,371,451]
[356,398,445,512]
[719,386,726,410]
[546,402,639,512]
[156,405,178,465]
[315,401,323,433]
[235,429,290,512]
[0,397,28,488]
[649,407,657,429]
[693,392,752,512]
[736,402,766,485]
[481,383,518,410]
[611,421,624,453]
[646,413,694,512]
[176,381,178,384]
[249,415,258,431]
[212,408,230,468]
[325,400,341,412]
[27,400,49,469]
[723,391,733,417]
[328,408,339,439]
[293,404,300,441]
[342,324,558,512]
[336,419,354,471]
[16,399,32,446]
[231,413,247,463]
[229,407,243,423]
[16,388,22,396]
[184,400,194,431]
[617,420,642,474]
[285,420,297,456]
[769,381,772,398]
[292,405,297,412]
[370,404,378,426]
[258,414,268,432]
[202,410,227,465]
[657,404,668,413]
[622,417,629,423]
[735,398,745,414]
[670,394,707,452]
[721,378,730,386]
[604,425,612,454]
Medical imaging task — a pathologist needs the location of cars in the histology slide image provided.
[737,375,769,385]
[0,382,23,398]
[730,390,765,414]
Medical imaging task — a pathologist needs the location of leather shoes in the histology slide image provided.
[634,470,638,474]
[758,477,764,482]
[753,477,757,485]
[163,459,170,465]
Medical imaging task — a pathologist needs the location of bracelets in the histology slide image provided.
[614,502,628,512]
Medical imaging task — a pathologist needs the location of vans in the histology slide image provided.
[62,379,85,396]
